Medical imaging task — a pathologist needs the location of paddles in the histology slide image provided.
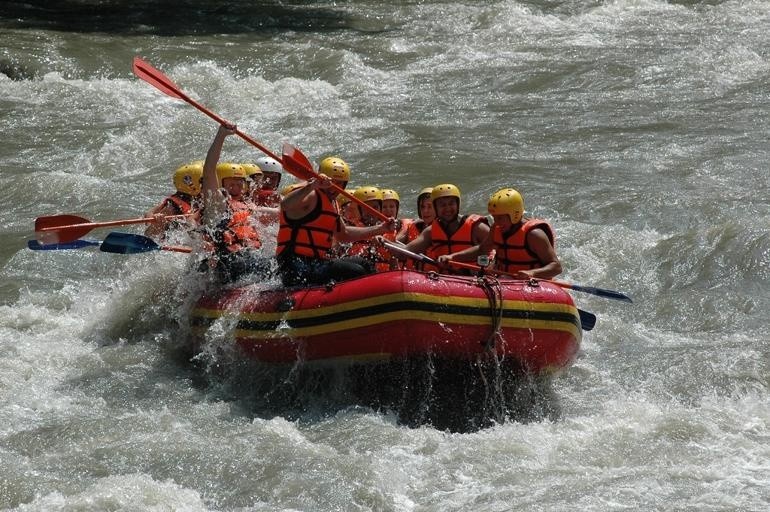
[384,241,595,331]
[34,214,191,245]
[28,239,100,250]
[100,231,194,253]
[283,144,403,231]
[447,259,631,303]
[133,55,282,163]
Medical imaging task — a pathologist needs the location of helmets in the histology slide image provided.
[216,163,246,188]
[172,160,206,196]
[352,187,382,217]
[257,157,283,174]
[488,188,524,224]
[318,157,350,182]
[380,189,400,215]
[242,164,263,176]
[337,189,356,207]
[431,183,461,213]
[417,188,433,218]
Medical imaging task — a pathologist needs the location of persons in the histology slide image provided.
[438,188,563,280]
[340,187,435,271]
[277,158,398,291]
[145,157,283,239]
[203,119,278,283]
[371,184,490,274]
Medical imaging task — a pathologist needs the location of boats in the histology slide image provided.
[190,275,581,378]
